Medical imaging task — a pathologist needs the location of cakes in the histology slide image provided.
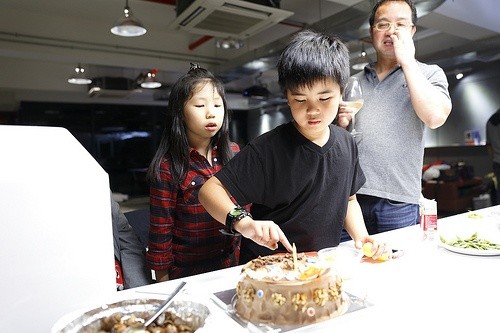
[235,253,346,323]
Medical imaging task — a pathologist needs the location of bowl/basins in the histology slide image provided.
[56,297,211,333]
[317,247,365,280]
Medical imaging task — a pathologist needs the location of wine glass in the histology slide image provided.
[340,76,364,136]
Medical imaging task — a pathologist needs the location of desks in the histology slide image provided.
[117,205,500,333]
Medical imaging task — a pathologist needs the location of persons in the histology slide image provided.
[146,63,252,284]
[198,31,392,266]
[485,107,500,206]
[110,190,152,290]
[336,0,452,242]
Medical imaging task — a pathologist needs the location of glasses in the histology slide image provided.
[372,20,414,32]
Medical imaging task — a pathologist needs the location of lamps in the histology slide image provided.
[109,0,148,38]
[67,65,93,84]
[136,69,163,89]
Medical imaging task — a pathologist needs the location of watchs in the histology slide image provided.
[219,208,252,237]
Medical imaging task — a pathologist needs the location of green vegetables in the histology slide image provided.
[439,232,500,251]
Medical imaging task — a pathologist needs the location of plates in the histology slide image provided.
[435,228,500,256]
